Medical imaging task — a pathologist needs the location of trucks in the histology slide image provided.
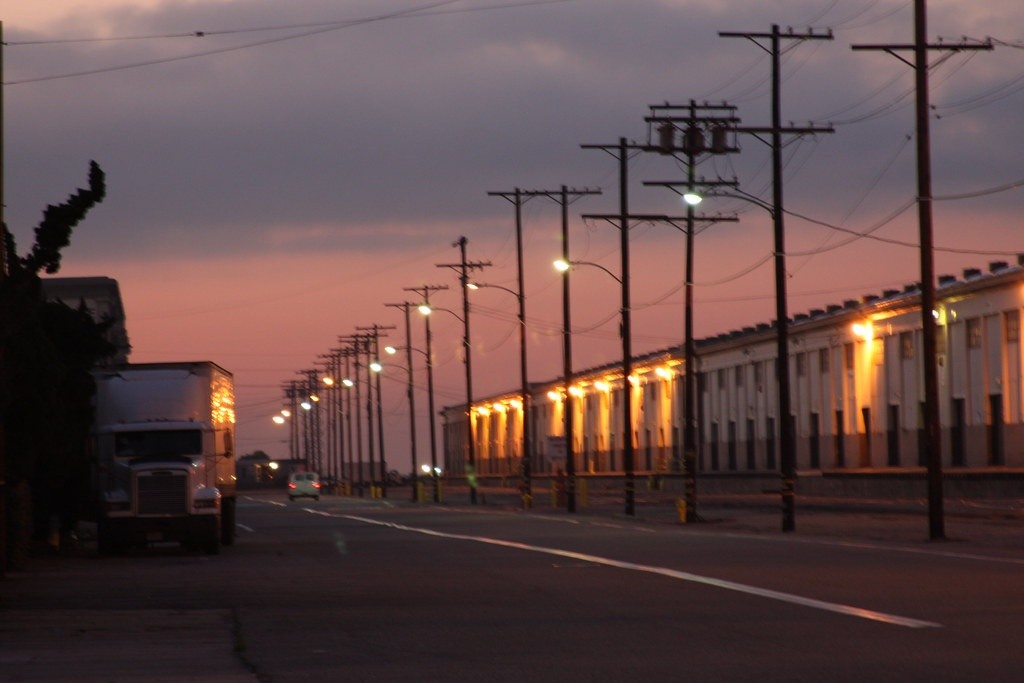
[86,362,237,555]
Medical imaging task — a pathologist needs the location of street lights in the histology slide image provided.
[554,257,634,516]
[466,282,535,509]
[273,346,440,504]
[683,192,795,533]
[418,305,477,506]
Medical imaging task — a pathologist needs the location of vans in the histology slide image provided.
[288,473,320,501]
